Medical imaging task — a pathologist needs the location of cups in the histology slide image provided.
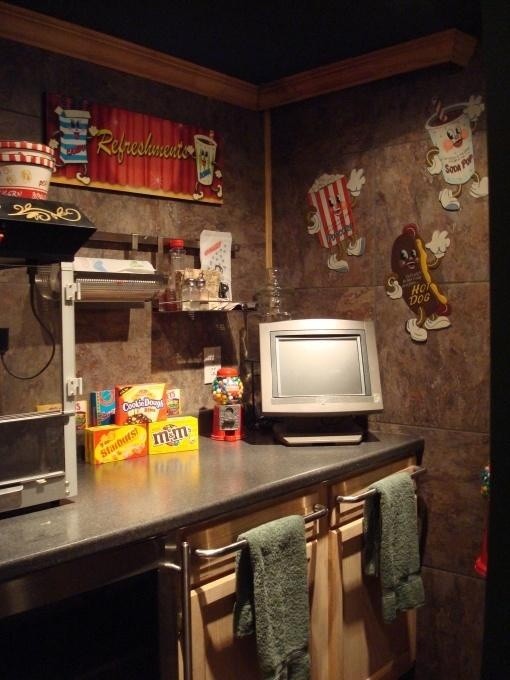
[424,102,475,185]
[193,134,218,186]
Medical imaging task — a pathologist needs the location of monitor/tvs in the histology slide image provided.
[260,317,384,447]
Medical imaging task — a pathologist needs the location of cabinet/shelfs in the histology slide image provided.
[178,457,417,680]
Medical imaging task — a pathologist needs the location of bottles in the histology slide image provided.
[211,368,244,405]
[196,276,209,311]
[161,239,188,311]
[182,278,197,312]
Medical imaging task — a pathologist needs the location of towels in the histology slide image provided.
[361,476,424,624]
[232,516,311,680]
